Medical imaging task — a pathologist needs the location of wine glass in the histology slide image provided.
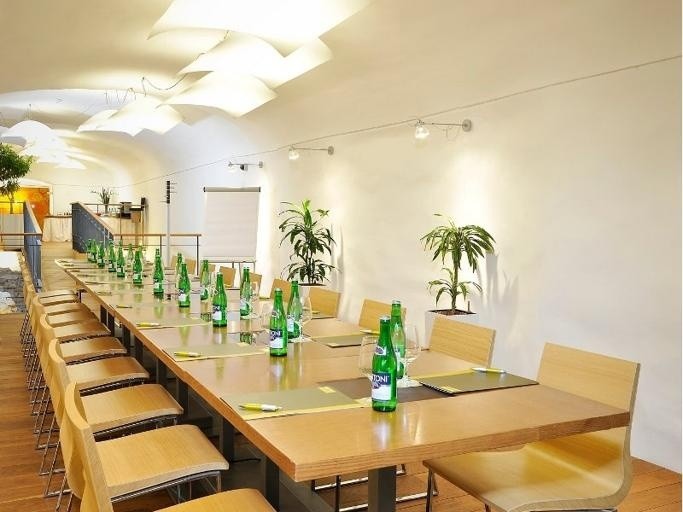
[240,281,421,407]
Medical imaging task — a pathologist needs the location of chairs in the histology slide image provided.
[421,343,640,512]
[311,316,496,512]
[358,299,406,334]
[170,254,294,305]
[305,286,341,317]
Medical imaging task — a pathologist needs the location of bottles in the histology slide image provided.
[153,249,165,297]
[212,272,227,326]
[177,253,190,307]
[87,241,144,288]
[199,258,211,303]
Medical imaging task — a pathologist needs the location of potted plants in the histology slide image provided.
[90,185,119,214]
[0,141,39,251]
[277,199,341,309]
[420,213,496,351]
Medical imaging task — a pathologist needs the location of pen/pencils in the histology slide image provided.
[311,311,320,314]
[471,367,506,373]
[174,351,203,356]
[77,273,89,276]
[136,322,161,327]
[63,263,74,266]
[116,304,132,308]
[259,296,270,299]
[238,403,282,412]
[85,281,98,283]
[224,284,232,287]
[70,269,80,272]
[359,329,381,335]
[98,291,112,294]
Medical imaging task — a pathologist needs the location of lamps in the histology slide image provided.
[155,72,282,118]
[176,32,335,88]
[109,94,184,135]
[146,0,372,57]
[77,108,142,137]
[414,119,472,139]
[228,161,263,172]
[1,120,63,142]
[288,146,334,160]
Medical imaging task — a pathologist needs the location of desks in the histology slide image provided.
[55,258,632,512]
[42,215,72,243]
